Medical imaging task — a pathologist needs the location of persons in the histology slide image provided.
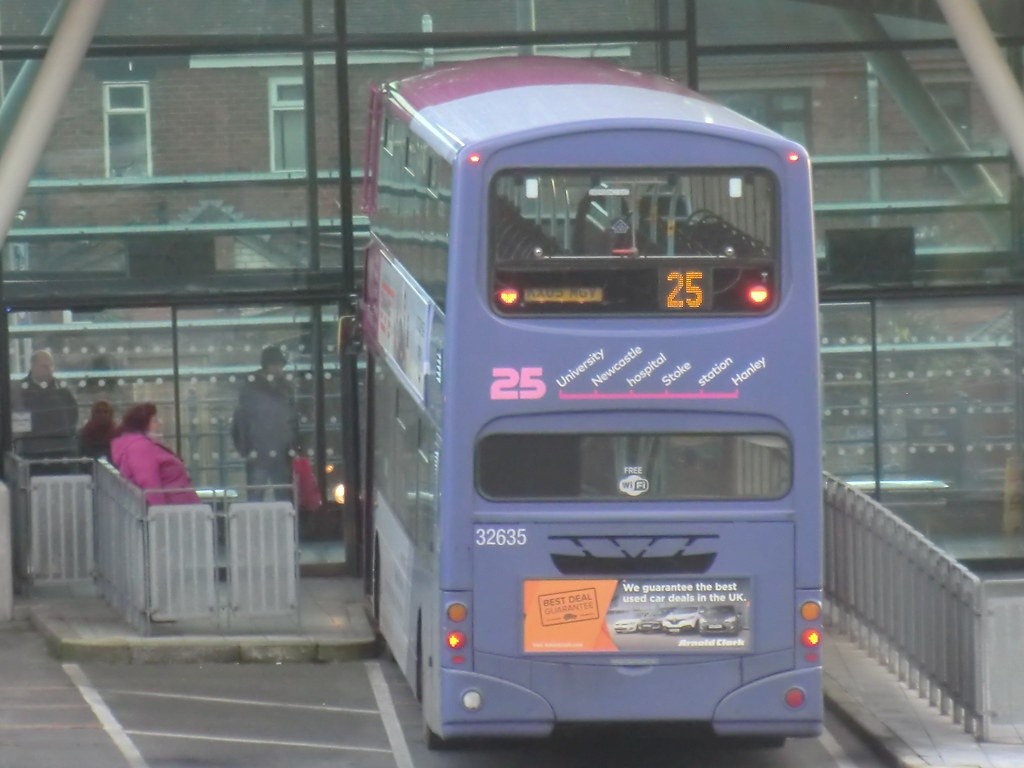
[233,346,302,510]
[22,350,200,509]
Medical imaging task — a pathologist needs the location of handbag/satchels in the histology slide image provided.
[292,451,323,510]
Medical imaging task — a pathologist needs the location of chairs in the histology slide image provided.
[677,210,770,262]
[494,195,595,313]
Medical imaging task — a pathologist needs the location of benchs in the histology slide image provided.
[851,481,949,541]
[195,489,239,544]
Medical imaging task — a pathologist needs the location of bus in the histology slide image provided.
[338,56,823,750]
